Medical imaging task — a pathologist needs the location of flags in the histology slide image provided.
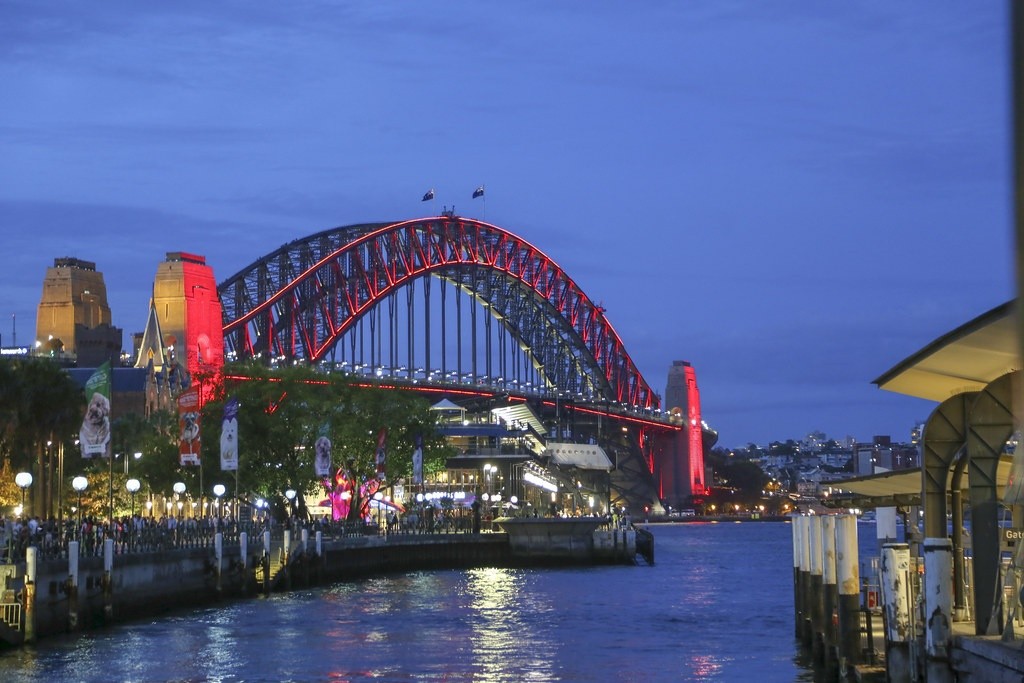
[472,186,485,198]
[422,189,433,201]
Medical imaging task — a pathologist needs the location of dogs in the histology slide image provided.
[413,447,423,483]
[315,437,332,477]
[79,393,110,458]
[375,445,387,478]
[180,410,201,465]
[220,418,238,470]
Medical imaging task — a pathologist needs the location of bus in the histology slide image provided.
[669,508,696,518]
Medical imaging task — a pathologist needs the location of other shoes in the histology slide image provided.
[376,528,438,535]
[601,525,632,532]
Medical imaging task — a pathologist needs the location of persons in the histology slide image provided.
[0,507,632,564]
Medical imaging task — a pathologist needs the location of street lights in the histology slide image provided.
[15,472,33,524]
[373,491,383,537]
[72,475,88,542]
[174,481,186,542]
[213,484,226,519]
[126,478,141,547]
[285,488,297,518]
